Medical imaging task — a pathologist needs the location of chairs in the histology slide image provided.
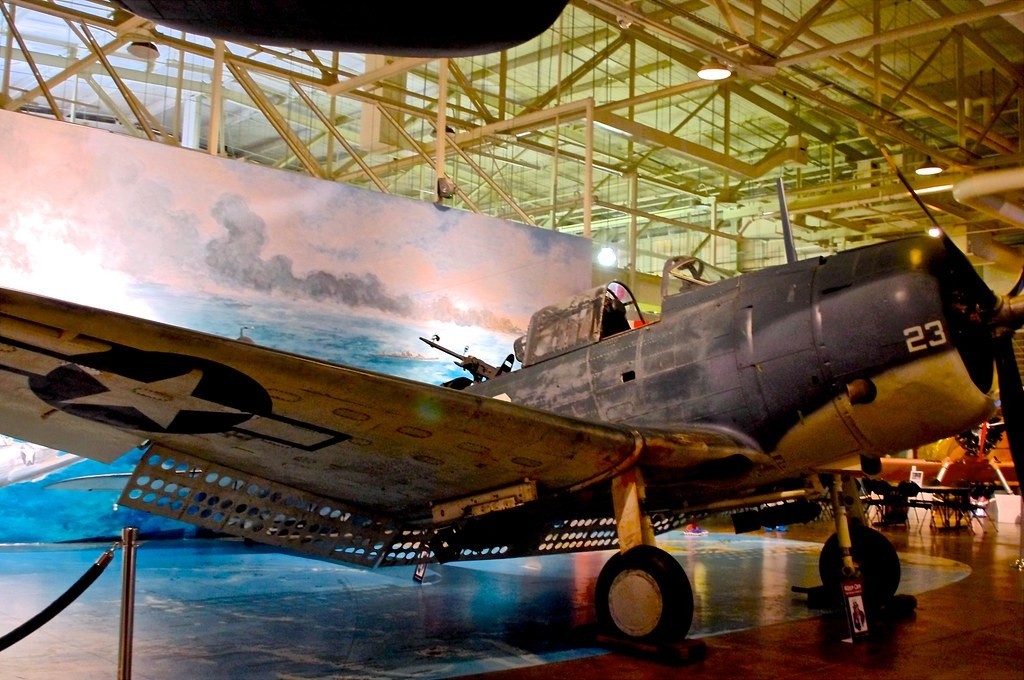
[814,498,834,522]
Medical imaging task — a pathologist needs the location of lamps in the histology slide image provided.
[696,36,732,82]
[915,143,943,176]
[127,41,160,60]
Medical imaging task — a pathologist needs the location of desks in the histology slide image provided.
[920,486,972,536]
[862,477,999,536]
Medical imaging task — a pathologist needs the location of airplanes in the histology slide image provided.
[0,121,1024,648]
